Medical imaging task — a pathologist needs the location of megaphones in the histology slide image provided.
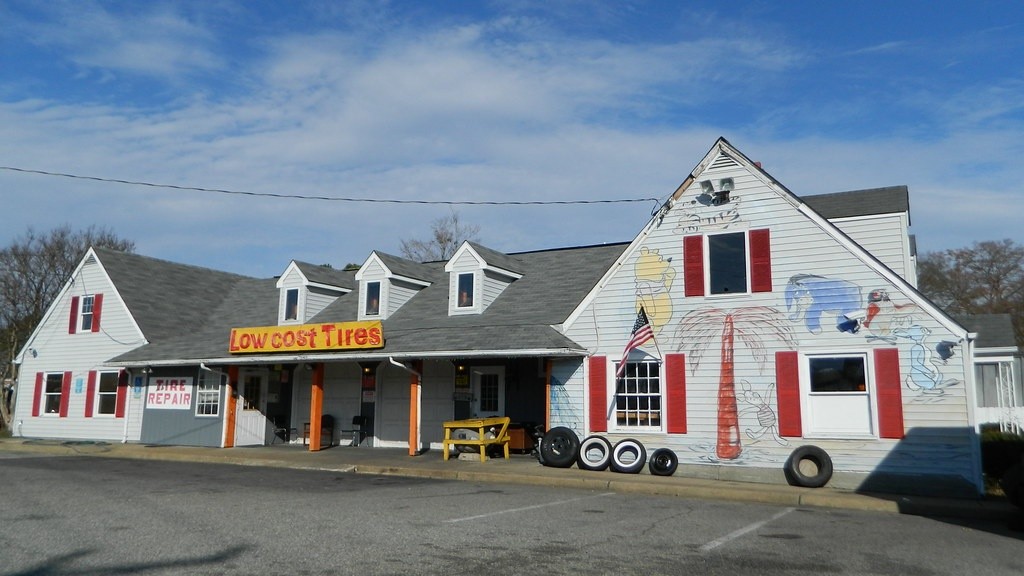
[700,177,734,196]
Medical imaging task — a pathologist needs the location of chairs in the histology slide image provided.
[303,413,333,448]
[268,414,299,446]
[338,415,371,449]
